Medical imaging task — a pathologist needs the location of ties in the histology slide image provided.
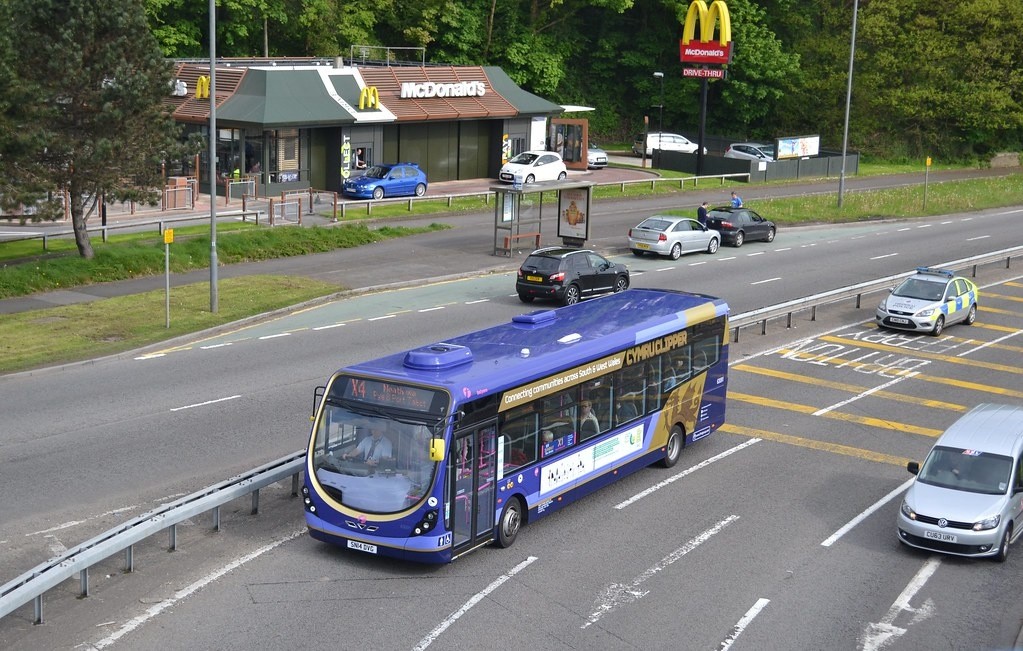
[367,439,376,460]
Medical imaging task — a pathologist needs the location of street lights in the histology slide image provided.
[652,72,664,167]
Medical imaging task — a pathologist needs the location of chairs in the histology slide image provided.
[500,348,708,462]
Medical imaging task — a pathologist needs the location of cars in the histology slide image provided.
[631,131,708,160]
[874,267,978,336]
[724,142,778,163]
[627,214,722,260]
[498,150,568,185]
[556,140,609,170]
[344,161,428,200]
[702,206,777,247]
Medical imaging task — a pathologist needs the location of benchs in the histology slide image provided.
[504,232,541,255]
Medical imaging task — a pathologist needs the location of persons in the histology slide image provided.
[731,191,745,209]
[582,400,601,434]
[615,400,634,422]
[352,149,367,168]
[250,161,261,173]
[937,450,969,480]
[342,428,393,466]
[663,373,676,391]
[543,430,554,443]
[697,202,710,224]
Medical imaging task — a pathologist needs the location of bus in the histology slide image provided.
[301,286,732,565]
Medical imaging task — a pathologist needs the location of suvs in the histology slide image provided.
[515,244,629,307]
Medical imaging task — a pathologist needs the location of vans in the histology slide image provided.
[896,401,1023,562]
